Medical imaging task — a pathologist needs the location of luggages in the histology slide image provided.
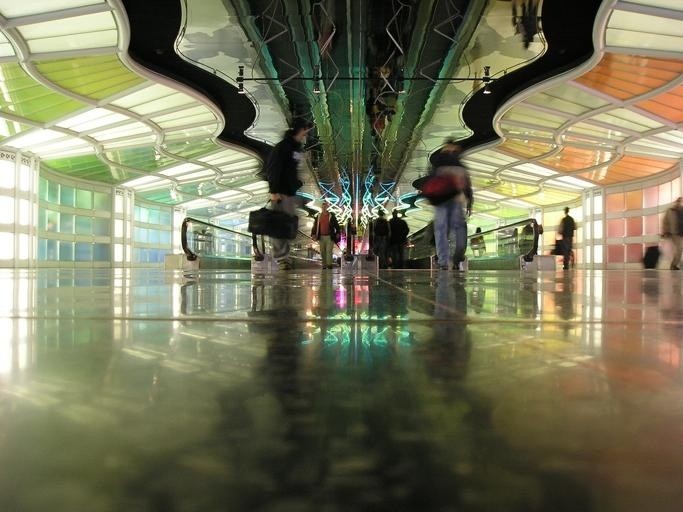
[644,247,660,268]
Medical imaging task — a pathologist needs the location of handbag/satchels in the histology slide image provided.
[248,208,297,240]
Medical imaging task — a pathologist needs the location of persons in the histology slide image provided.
[429,137,474,273]
[265,116,315,275]
[311,201,340,270]
[557,206,575,270]
[659,196,683,270]
[471,227,486,258]
[372,209,409,269]
